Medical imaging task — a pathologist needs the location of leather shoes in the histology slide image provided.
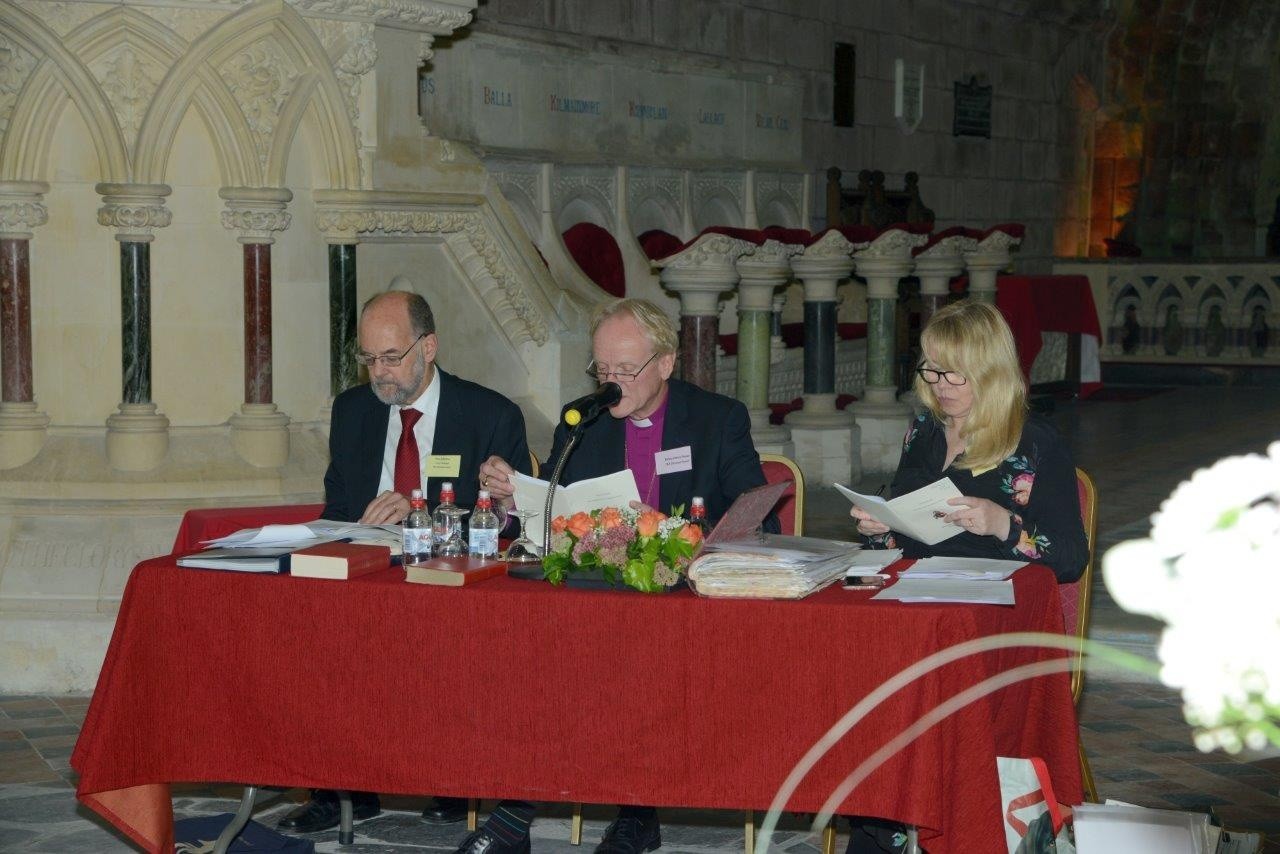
[419,797,482,821]
[455,826,530,854]
[277,793,383,835]
[594,810,661,854]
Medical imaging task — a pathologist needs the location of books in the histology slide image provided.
[290,541,392,580]
[405,554,507,587]
[507,469,643,549]
[833,477,972,546]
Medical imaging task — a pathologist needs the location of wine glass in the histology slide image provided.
[472,501,507,559]
[505,509,540,561]
[438,509,469,557]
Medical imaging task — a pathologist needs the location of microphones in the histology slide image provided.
[564,381,622,425]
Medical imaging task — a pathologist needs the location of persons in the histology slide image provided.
[273,291,534,833]
[845,300,1091,854]
[452,297,781,854]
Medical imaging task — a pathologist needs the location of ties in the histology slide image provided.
[394,408,424,503]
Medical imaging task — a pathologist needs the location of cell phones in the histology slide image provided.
[842,575,884,591]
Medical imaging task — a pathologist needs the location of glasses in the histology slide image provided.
[354,332,427,368]
[586,351,662,382]
[916,359,969,387]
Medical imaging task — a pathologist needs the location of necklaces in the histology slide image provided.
[625,439,661,504]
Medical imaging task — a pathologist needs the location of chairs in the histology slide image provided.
[304,449,1096,853]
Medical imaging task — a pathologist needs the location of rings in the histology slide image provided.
[482,475,489,487]
[968,518,974,527]
[393,504,396,512]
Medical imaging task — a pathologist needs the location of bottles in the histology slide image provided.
[432,482,461,557]
[403,489,433,570]
[688,495,715,540]
[469,490,499,559]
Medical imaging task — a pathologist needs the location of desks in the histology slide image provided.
[952,274,1102,407]
[70,559,1085,854]
[171,502,325,555]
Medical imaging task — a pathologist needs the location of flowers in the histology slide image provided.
[540,510,709,595]
